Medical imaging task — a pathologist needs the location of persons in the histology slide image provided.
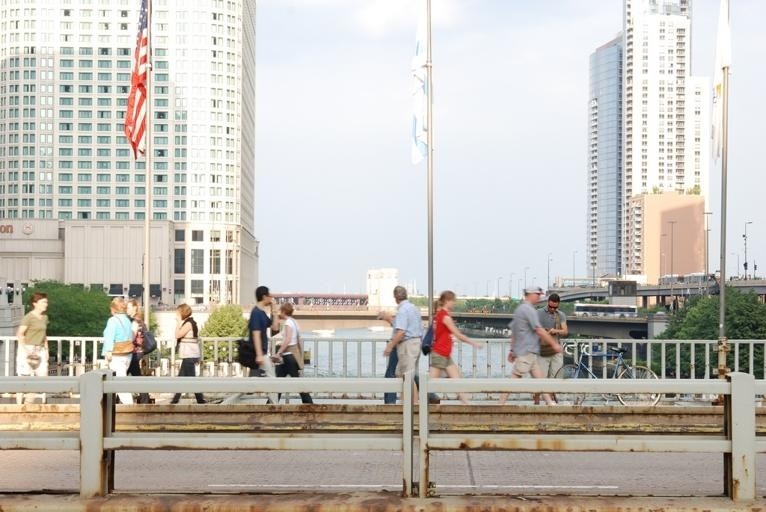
[15,292,50,404]
[499,287,562,406]
[116,298,150,404]
[382,286,424,404]
[536,293,569,379]
[266,302,313,403]
[100,296,135,405]
[169,304,207,403]
[377,310,441,404]
[428,289,483,405]
[220,286,279,405]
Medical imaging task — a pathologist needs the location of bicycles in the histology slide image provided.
[553,344,663,406]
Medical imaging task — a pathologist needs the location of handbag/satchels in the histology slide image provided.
[143,332,156,354]
[539,328,560,357]
[112,340,135,354]
[178,337,201,359]
[238,340,259,369]
[422,325,432,355]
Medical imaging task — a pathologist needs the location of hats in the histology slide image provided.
[524,287,544,294]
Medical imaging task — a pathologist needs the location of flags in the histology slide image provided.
[410,15,434,166]
[123,0,149,161]
[710,0,733,165]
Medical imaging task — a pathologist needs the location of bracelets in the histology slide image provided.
[510,349,514,352]
[278,351,282,355]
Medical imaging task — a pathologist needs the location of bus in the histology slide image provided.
[574,303,638,319]
[662,273,706,283]
